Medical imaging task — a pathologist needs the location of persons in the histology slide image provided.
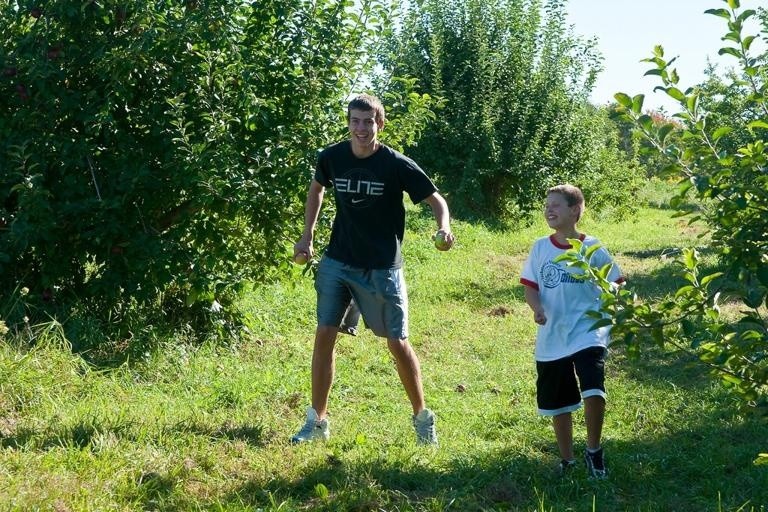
[515,182,628,486]
[287,92,455,449]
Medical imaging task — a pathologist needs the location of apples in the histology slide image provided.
[435,234,452,251]
[295,252,308,264]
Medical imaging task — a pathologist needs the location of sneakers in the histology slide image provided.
[291,406,329,443]
[584,447,609,481]
[411,408,438,446]
[557,457,575,474]
